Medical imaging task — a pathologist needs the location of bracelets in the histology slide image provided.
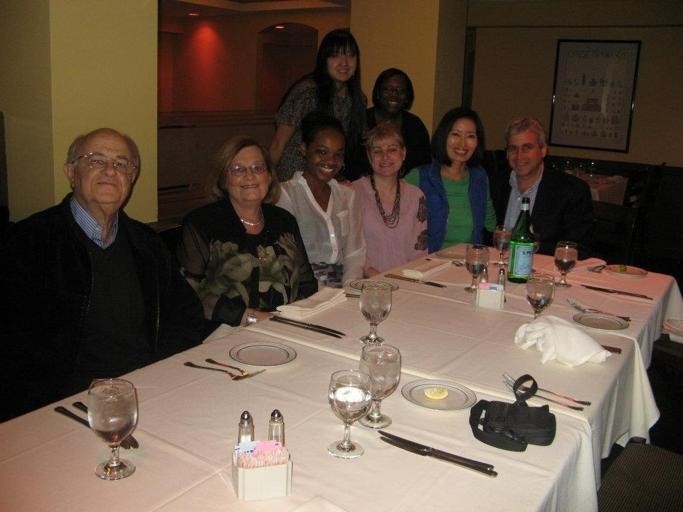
[246,308,257,330]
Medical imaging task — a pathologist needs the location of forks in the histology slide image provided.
[184,361,257,381]
[503,380,583,413]
[566,299,632,324]
[503,372,592,406]
[205,358,267,377]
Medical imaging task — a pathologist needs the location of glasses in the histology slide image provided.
[381,88,407,98]
[228,160,267,175]
[71,151,138,174]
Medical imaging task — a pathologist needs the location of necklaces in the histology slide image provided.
[234,206,265,227]
[368,174,402,229]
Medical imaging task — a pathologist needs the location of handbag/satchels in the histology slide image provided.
[470,375,556,452]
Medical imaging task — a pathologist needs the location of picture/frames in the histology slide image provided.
[547,38,641,152]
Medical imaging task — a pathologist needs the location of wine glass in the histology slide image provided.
[492,224,515,269]
[327,369,373,459]
[465,245,490,292]
[531,233,541,275]
[554,242,578,288]
[86,377,139,479]
[358,281,393,347]
[526,272,556,322]
[355,345,402,427]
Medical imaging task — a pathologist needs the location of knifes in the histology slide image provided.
[384,273,447,287]
[273,315,346,337]
[54,405,130,450]
[269,318,342,339]
[579,283,654,301]
[72,401,140,450]
[381,436,498,478]
[377,430,493,469]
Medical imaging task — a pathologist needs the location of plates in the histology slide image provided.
[603,264,649,279]
[349,277,401,293]
[572,311,629,332]
[400,378,477,411]
[228,342,297,366]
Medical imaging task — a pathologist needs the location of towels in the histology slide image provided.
[515,315,613,368]
[404,257,451,277]
[553,259,606,274]
[276,286,346,317]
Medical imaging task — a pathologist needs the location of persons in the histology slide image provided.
[266,27,369,192]
[0,128,207,424]
[486,117,591,255]
[344,68,433,180]
[274,111,366,292]
[173,136,320,329]
[404,105,498,253]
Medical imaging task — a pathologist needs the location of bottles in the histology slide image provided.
[237,411,255,443]
[497,269,507,302]
[269,409,285,444]
[506,195,535,285]
[480,267,489,285]
